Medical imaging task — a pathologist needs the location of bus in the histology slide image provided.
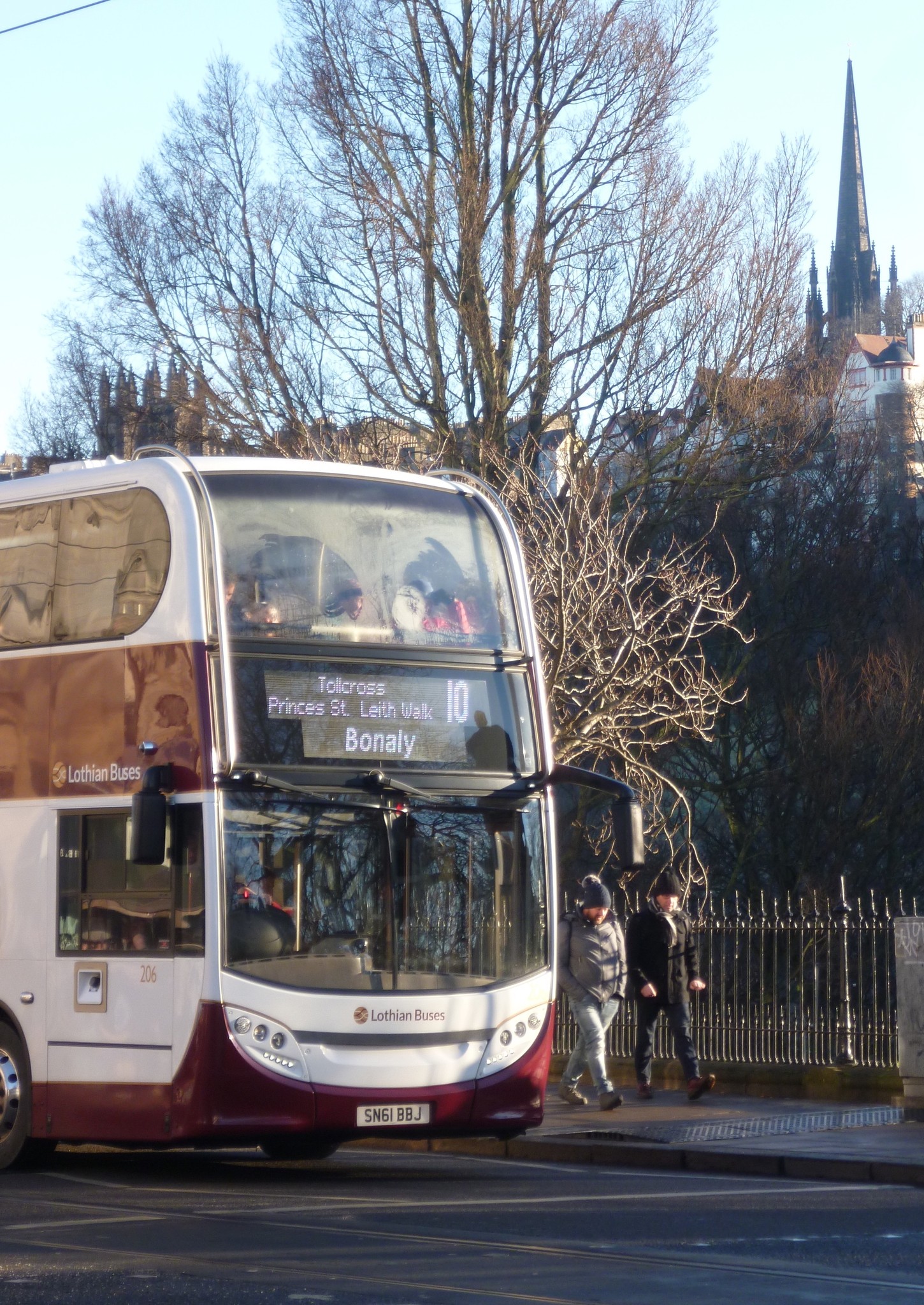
[0,440,646,1173]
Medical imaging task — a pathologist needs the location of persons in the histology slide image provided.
[557,874,628,1110]
[626,866,716,1101]
[214,562,483,645]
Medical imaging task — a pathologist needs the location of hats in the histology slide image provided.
[580,874,612,910]
[654,866,681,897]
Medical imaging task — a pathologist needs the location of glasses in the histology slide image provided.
[662,894,678,899]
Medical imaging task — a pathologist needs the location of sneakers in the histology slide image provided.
[689,1075,715,1102]
[636,1084,653,1099]
[558,1086,588,1105]
[598,1092,624,1112]
